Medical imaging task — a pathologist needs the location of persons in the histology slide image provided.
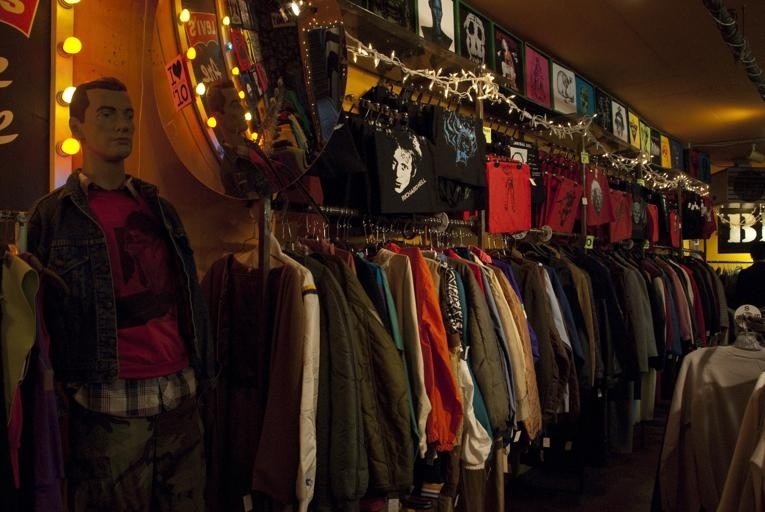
[381,137,421,198]
[735,238,765,307]
[20,73,213,510]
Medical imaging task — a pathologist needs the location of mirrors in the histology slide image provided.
[149,0,348,202]
